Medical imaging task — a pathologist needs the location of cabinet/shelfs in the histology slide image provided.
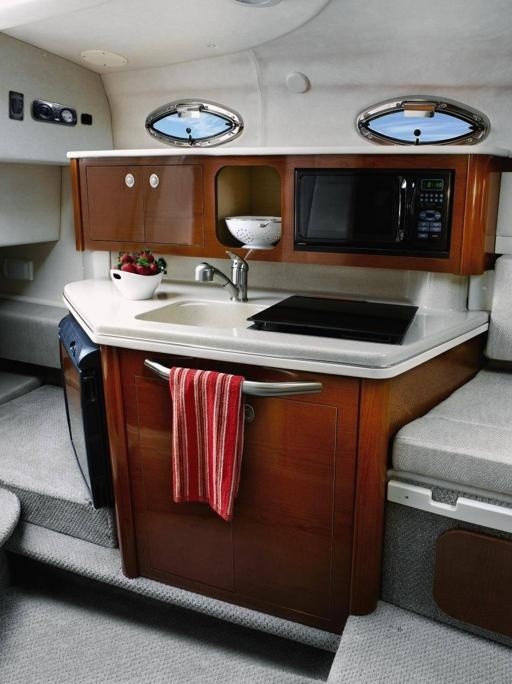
[77,157,205,256]
[99,333,490,633]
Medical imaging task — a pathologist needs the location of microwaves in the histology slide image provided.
[291,165,456,259]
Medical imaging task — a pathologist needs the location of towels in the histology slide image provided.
[168,366,245,524]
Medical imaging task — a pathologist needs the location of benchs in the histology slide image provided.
[0,374,117,550]
[380,252,511,649]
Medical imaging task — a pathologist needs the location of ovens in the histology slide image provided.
[58,312,116,511]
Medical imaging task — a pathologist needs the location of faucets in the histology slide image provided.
[195,251,249,302]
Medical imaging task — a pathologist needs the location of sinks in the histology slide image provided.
[135,300,270,324]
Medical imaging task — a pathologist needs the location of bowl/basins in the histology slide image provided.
[106,270,164,301]
[222,214,282,250]
